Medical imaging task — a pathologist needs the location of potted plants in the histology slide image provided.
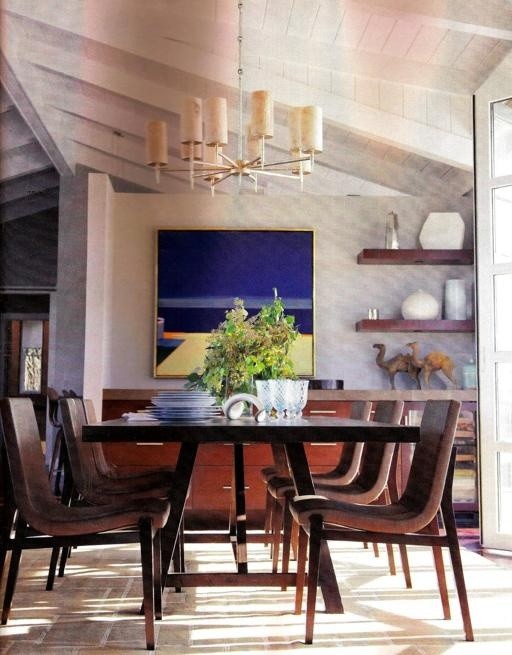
[185,288,309,419]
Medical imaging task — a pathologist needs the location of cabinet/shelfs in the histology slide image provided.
[355,248,474,332]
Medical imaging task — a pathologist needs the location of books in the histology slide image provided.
[402,406,477,504]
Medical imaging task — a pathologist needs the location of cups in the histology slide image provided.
[367,307,380,320]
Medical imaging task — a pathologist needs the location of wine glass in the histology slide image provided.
[255,378,309,424]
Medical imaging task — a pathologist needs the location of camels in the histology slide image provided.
[373,343,421,390]
[406,342,459,390]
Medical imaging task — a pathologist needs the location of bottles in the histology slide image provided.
[445,279,466,321]
[386,213,399,249]
[462,358,478,390]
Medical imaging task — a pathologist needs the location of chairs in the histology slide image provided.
[1,397,172,651]
[45,397,182,591]
[69,400,176,559]
[271,400,412,591]
[296,399,474,645]
[260,400,372,558]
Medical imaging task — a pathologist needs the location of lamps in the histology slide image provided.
[146,0,324,198]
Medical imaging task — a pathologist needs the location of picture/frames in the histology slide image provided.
[151,226,316,380]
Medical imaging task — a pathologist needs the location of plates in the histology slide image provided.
[137,390,225,421]
